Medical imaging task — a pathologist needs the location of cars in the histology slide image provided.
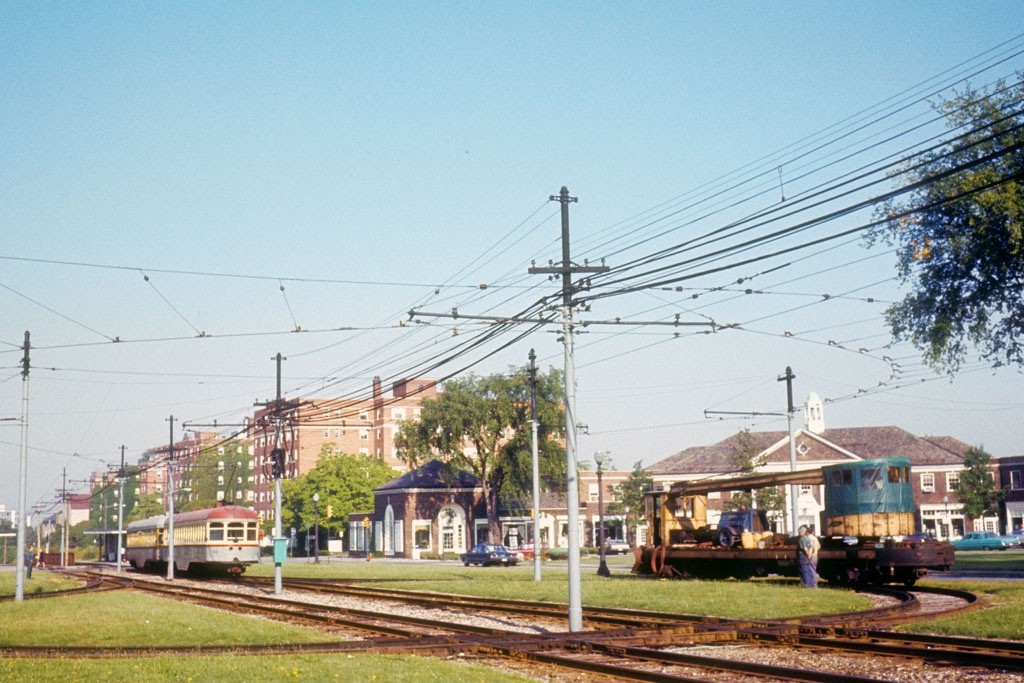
[459,542,517,568]
[598,540,630,555]
[949,532,1021,551]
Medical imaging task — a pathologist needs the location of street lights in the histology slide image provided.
[594,451,612,578]
[313,493,320,564]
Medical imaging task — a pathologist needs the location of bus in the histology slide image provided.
[644,458,956,593]
[127,468,266,580]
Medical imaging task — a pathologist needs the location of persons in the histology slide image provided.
[36,548,46,569]
[799,526,821,588]
[26,545,34,579]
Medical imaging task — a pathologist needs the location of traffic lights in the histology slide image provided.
[363,518,370,528]
[327,504,334,518]
